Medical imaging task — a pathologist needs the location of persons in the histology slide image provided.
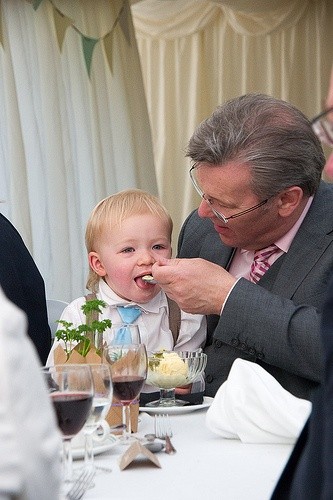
[43,187,209,403]
[151,93,333,500]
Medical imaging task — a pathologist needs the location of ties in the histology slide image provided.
[111,305,145,348]
[241,242,280,288]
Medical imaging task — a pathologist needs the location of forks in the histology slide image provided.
[63,470,95,500]
[153,412,177,456]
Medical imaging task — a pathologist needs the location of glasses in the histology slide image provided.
[188,160,283,225]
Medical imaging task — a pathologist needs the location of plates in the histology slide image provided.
[61,435,120,461]
[139,398,214,416]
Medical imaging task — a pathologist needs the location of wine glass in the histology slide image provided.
[73,364,112,477]
[40,362,94,491]
[103,344,149,444]
[98,323,142,351]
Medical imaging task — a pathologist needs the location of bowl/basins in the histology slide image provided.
[145,349,207,406]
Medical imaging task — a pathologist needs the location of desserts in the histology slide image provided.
[148,351,190,386]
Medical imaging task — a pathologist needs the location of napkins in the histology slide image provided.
[206,358,301,446]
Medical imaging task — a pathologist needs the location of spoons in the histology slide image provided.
[141,275,157,286]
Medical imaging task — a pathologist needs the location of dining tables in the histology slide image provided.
[62,382,312,500]
